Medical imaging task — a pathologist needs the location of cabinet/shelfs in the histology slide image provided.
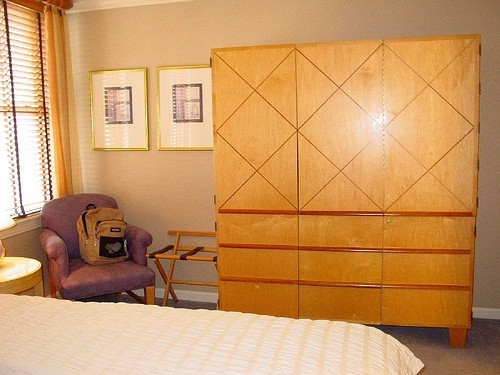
[212,33,481,346]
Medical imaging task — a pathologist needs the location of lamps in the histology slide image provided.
[0,203,16,264]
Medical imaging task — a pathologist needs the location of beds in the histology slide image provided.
[0,294,424,375]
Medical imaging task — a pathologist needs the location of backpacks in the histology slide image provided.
[76,204,128,265]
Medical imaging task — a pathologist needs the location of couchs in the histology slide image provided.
[39,193,155,305]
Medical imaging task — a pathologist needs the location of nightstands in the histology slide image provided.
[0,257,43,297]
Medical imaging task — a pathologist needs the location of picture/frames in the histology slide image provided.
[89,64,214,151]
